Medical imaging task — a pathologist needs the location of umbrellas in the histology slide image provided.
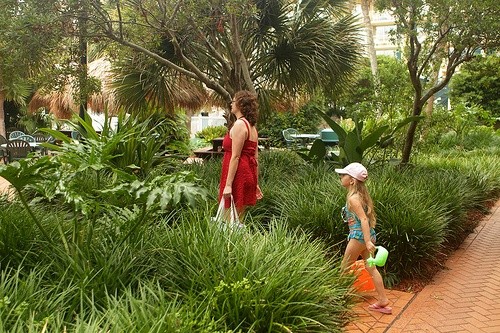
[28,56,205,120]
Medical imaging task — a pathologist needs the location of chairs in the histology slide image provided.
[0,130,53,163]
[282,127,302,150]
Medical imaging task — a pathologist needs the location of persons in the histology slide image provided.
[218,89,264,225]
[335,162,392,314]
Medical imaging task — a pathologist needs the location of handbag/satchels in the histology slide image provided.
[211,195,247,235]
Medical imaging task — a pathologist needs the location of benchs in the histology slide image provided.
[194,146,226,155]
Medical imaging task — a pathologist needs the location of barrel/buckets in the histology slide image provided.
[351,261,374,293]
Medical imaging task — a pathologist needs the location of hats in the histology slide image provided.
[335,162,369,183]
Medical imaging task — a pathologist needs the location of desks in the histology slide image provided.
[290,134,319,147]
[211,138,268,151]
[0,141,43,162]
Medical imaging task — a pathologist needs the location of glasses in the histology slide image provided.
[229,100,236,104]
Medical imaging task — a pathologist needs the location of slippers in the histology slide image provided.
[368,304,392,313]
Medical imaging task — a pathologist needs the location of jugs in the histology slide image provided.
[368,245,389,268]
